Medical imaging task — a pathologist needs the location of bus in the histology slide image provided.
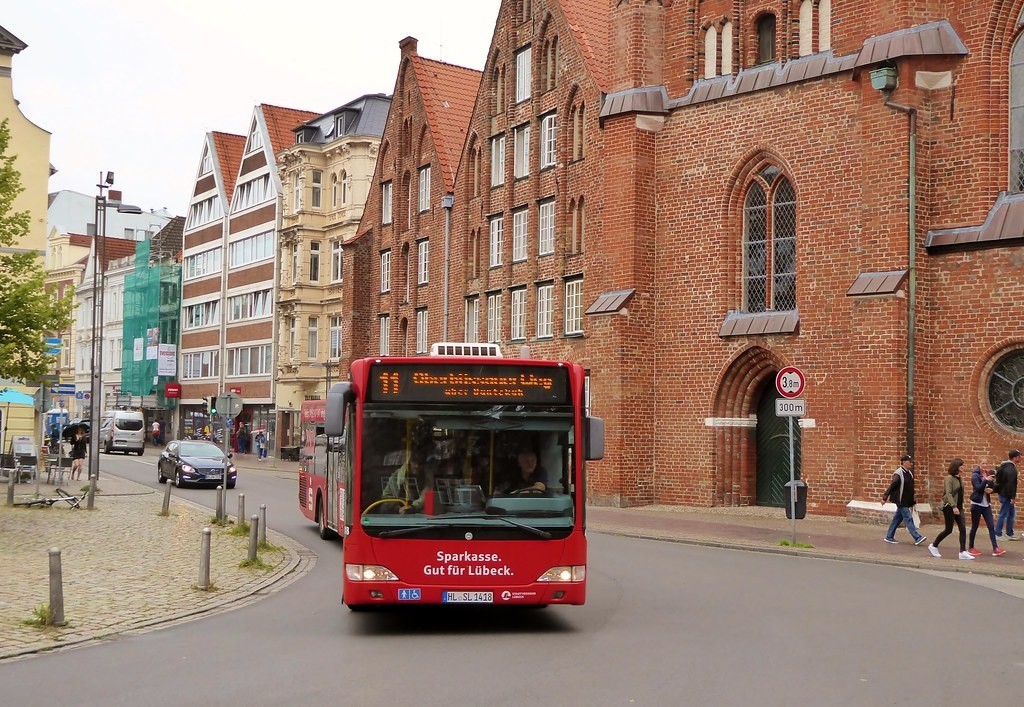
[296,338,607,608]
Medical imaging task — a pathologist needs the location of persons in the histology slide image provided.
[493,451,549,493]
[966,456,1006,557]
[151,418,167,446]
[381,453,436,514]
[881,455,927,546]
[69,427,88,481]
[927,459,976,560]
[235,421,268,460]
[993,450,1021,541]
[49,423,59,445]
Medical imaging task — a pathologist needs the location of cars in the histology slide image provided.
[63,418,92,440]
[157,439,237,487]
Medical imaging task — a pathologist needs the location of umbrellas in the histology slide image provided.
[0,388,35,476]
[250,429,265,433]
[62,420,90,439]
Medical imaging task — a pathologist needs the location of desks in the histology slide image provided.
[44,459,59,481]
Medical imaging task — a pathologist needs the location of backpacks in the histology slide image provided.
[993,464,1013,493]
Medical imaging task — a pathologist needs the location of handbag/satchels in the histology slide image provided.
[905,505,920,533]
[260,442,266,449]
[940,502,951,513]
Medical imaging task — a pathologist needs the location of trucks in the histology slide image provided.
[97,410,146,456]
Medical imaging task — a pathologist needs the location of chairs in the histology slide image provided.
[48,456,73,485]
[13,456,38,483]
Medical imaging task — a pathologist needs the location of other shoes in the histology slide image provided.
[994,535,1008,542]
[1005,533,1020,540]
[258,456,262,461]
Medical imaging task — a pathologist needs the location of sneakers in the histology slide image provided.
[993,547,1006,556]
[883,536,900,544]
[958,550,975,560]
[927,543,941,558]
[967,547,982,556]
[915,536,927,545]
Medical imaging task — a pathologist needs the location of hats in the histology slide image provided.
[901,455,915,461]
[1009,449,1021,458]
